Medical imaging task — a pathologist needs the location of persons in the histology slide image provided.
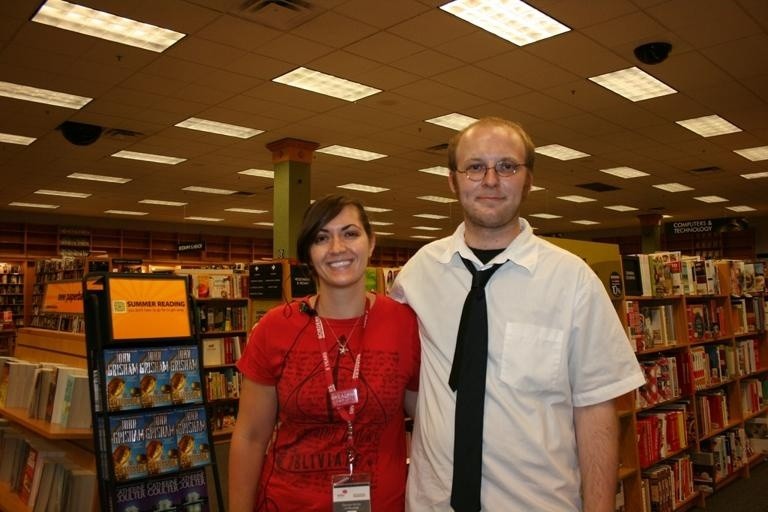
[227,193,422,512]
[388,117,647,512]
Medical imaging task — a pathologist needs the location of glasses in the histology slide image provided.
[454,160,527,181]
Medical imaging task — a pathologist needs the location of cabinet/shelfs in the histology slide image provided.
[0,224,768,512]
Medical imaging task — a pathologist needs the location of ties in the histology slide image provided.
[450,255,510,512]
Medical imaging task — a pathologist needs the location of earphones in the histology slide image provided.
[298,299,317,315]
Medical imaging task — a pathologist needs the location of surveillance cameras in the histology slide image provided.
[633,41,675,65]
[61,122,101,146]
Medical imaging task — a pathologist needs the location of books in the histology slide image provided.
[364,268,403,292]
[1,257,250,511]
[614,250,767,511]
